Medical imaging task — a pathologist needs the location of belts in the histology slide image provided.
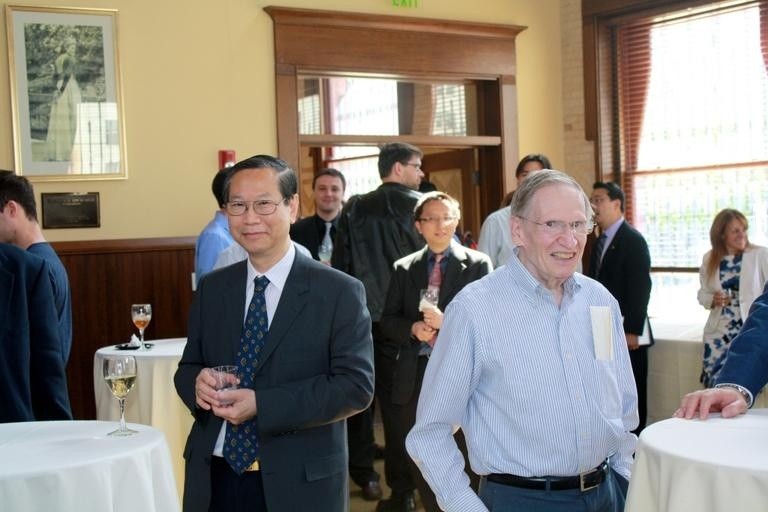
[243,459,261,473]
[487,460,611,494]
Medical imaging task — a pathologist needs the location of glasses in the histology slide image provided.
[419,215,457,227]
[407,163,419,170]
[516,215,595,235]
[226,199,285,217]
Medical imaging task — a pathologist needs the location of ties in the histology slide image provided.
[222,275,271,479]
[424,254,444,347]
[319,222,333,264]
[593,232,607,277]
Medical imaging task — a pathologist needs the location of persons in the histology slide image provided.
[168,137,658,510]
[0,240,75,423]
[43,36,84,168]
[693,205,768,388]
[0,168,76,374]
[669,278,768,421]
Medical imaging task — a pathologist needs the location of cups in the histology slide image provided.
[417,288,439,310]
[208,365,238,407]
[318,244,331,262]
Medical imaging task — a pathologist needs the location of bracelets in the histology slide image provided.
[713,382,751,404]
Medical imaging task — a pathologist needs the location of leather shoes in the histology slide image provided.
[375,490,416,512]
[362,480,383,500]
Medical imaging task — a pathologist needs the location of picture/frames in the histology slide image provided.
[4,3,129,181]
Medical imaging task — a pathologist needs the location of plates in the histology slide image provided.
[114,343,154,351]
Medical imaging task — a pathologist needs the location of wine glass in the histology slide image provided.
[130,303,153,348]
[101,356,140,438]
[715,288,736,318]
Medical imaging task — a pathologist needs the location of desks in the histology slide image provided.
[622,408,767,512]
